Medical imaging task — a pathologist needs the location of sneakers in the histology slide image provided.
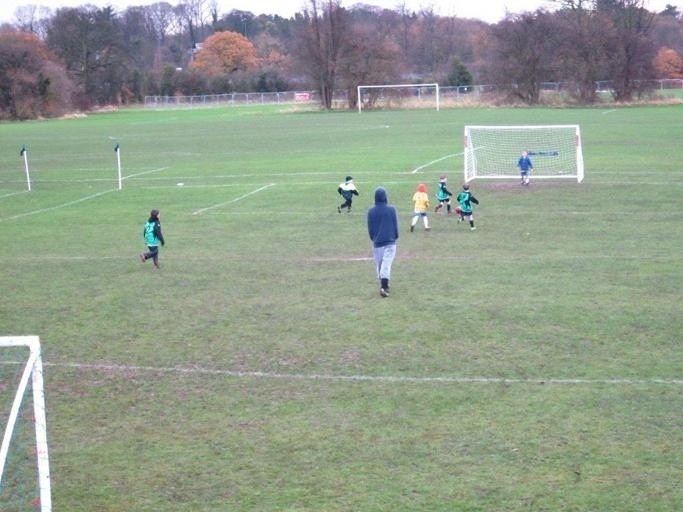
[140,254,146,262]
[470,226,476,230]
[380,283,390,297]
[337,207,351,214]
[410,226,414,232]
[521,181,529,186]
[457,218,462,224]
[154,261,160,268]
[425,226,431,230]
[435,207,452,214]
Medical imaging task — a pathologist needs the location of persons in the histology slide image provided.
[410,183,432,232]
[368,186,399,297]
[139,208,165,268]
[457,182,479,232]
[337,175,360,213]
[434,175,453,216]
[517,151,533,185]
